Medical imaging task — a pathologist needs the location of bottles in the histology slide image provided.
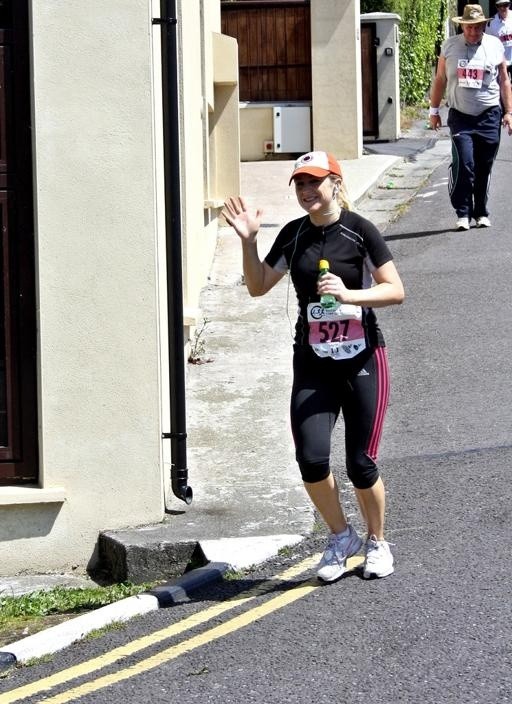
[317,258,337,308]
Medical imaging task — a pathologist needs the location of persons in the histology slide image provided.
[429,4,512,230]
[489,0,512,88]
[220,151,405,581]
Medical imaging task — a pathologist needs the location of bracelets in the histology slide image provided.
[429,106,439,116]
[505,112,512,116]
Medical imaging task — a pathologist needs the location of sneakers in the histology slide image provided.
[316,526,394,582]
[455,216,491,231]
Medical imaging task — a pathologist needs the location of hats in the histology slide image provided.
[452,4,493,24]
[289,151,342,186]
